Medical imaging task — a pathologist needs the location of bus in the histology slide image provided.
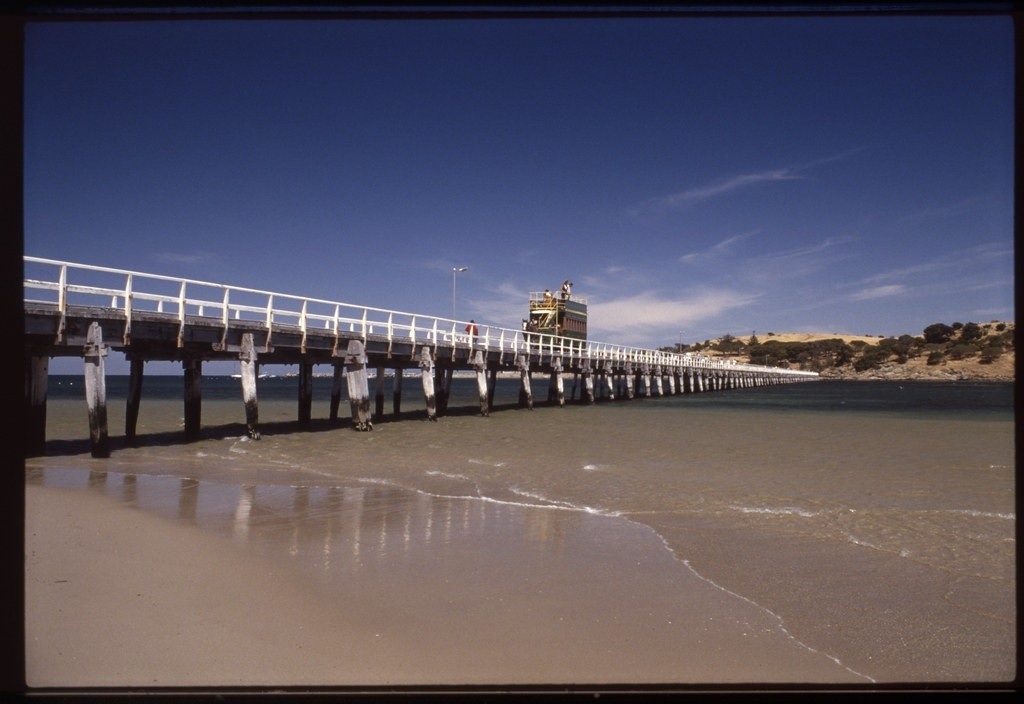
[522,289,587,348]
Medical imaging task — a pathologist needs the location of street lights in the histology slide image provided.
[453,266,468,321]
[739,347,744,362]
[680,330,685,353]
[766,354,770,365]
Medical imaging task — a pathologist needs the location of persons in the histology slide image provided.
[544,289,552,301]
[654,348,737,366]
[562,280,573,300]
[465,319,478,343]
